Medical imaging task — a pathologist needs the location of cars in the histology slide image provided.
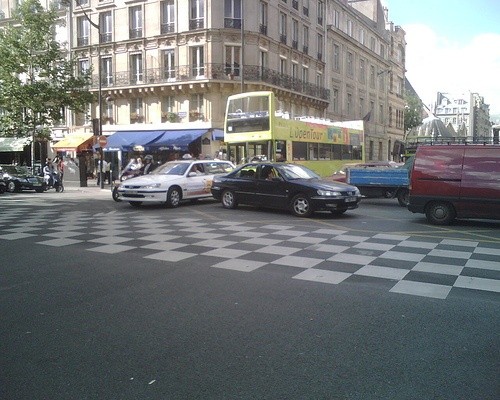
[0,164,65,192]
[211,163,361,217]
[112,160,235,207]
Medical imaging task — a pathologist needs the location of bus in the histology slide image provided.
[224,91,366,179]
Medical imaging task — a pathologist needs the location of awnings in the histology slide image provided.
[0,137,31,152]
[52,133,96,153]
[101,130,210,153]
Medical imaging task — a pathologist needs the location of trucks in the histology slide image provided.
[347,156,415,207]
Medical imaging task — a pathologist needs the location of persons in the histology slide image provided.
[42,147,267,184]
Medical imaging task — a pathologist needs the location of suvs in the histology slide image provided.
[410,145,500,227]
